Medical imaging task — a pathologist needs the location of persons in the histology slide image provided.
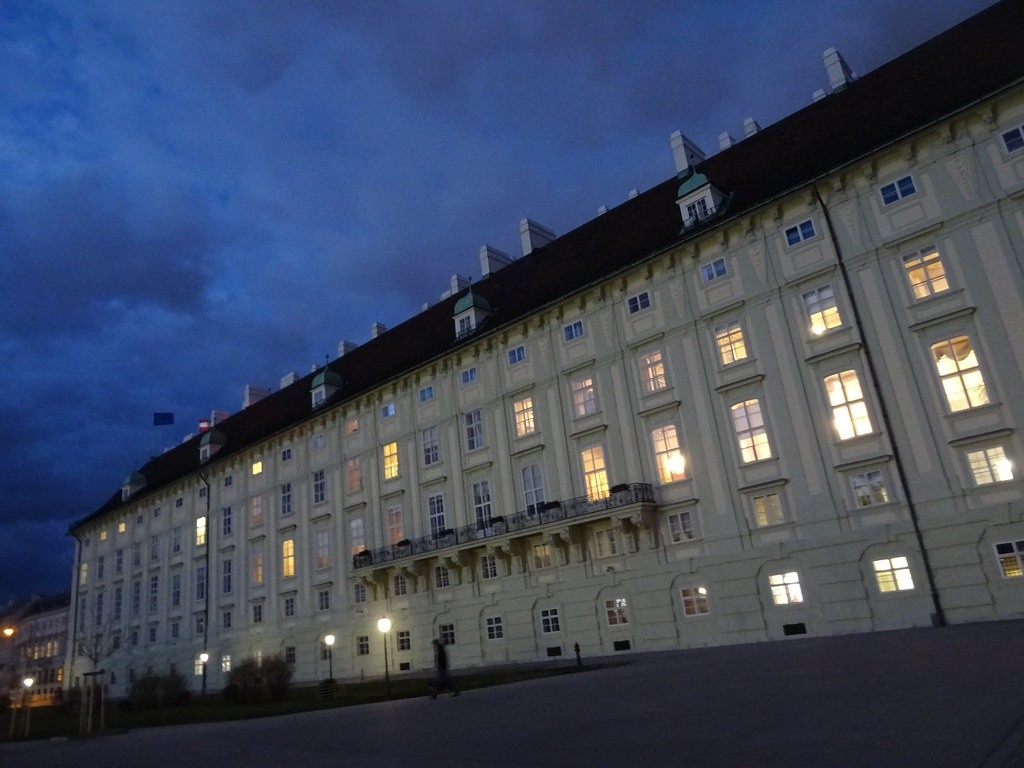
[429,637,461,701]
